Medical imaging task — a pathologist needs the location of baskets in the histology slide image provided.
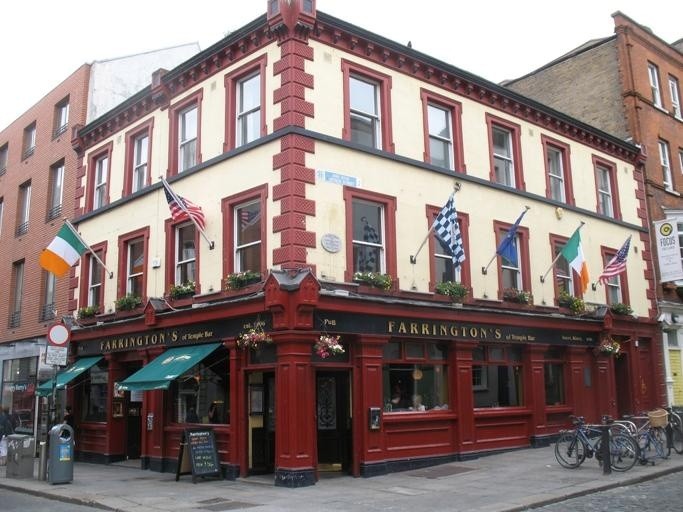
[648,408,670,427]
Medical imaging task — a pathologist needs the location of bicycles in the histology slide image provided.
[554,406,683,477]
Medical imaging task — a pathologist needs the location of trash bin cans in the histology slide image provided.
[48,424,75,484]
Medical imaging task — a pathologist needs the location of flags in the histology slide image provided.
[163,183,205,231]
[561,230,590,295]
[599,236,631,287]
[433,195,465,270]
[133,253,144,286]
[652,216,682,284]
[38,220,88,280]
[240,207,261,232]
[495,211,526,269]
[357,222,379,271]
[556,268,567,291]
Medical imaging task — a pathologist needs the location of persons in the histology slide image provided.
[389,393,402,409]
[408,394,426,411]
[60,405,76,439]
[187,402,230,424]
[1,407,17,466]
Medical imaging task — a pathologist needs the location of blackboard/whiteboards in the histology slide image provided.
[189,432,218,474]
